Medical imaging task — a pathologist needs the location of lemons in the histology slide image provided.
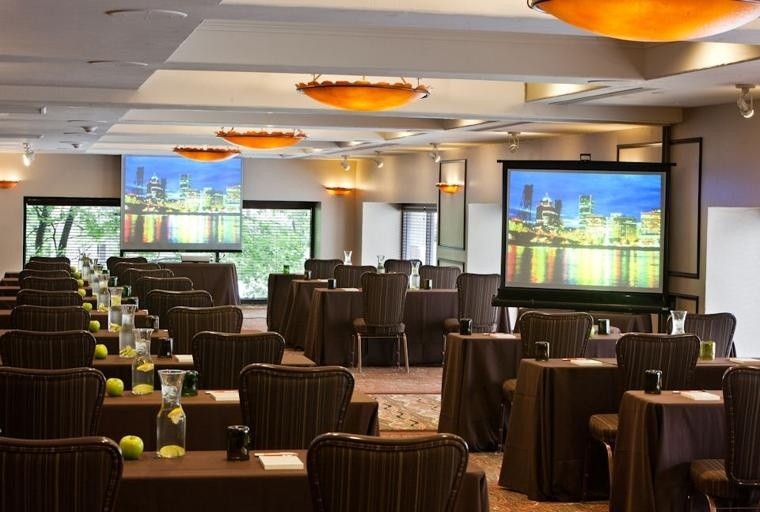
[111,297,120,305]
[160,445,185,457]
[168,408,182,424]
[133,384,153,395]
[137,362,154,372]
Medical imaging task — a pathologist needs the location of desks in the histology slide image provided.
[497,357,760,503]
[0,277,19,286]
[5,272,20,278]
[122,452,488,512]
[0,296,126,309]
[266,273,305,333]
[159,262,241,306]
[438,332,621,453]
[93,354,193,390]
[0,329,171,355]
[99,383,380,452]
[279,280,329,350]
[0,286,20,295]
[609,389,724,512]
[0,309,147,329]
[304,288,510,367]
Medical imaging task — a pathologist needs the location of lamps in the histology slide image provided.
[735,84,755,119]
[322,185,353,197]
[174,145,240,163]
[526,1,759,42]
[0,172,23,189]
[22,144,37,165]
[436,183,459,193]
[506,132,520,152]
[373,151,384,168]
[216,129,307,151]
[341,155,351,171]
[427,143,440,161]
[296,72,430,112]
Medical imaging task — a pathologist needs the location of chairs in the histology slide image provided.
[29,256,70,264]
[24,261,71,272]
[191,331,286,391]
[18,270,70,285]
[581,333,701,502]
[418,265,461,290]
[495,311,593,452]
[0,366,106,438]
[690,367,760,512]
[304,259,343,279]
[9,305,91,331]
[334,265,377,289]
[666,313,737,359]
[114,262,161,280]
[441,272,503,366]
[143,289,213,329]
[350,271,410,373]
[239,361,355,450]
[20,277,78,291]
[166,305,243,355]
[131,276,193,298]
[384,259,422,279]
[307,432,471,512]
[106,256,147,270]
[122,268,174,286]
[17,289,83,306]
[0,329,97,370]
[0,437,124,512]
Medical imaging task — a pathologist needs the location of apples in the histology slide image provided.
[119,435,143,459]
[82,302,92,312]
[94,344,107,359]
[77,279,84,287]
[71,265,76,272]
[78,289,86,297]
[89,320,100,333]
[73,272,80,279]
[105,378,124,397]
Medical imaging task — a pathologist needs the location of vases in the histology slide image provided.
[97,274,110,312]
[156,369,187,460]
[670,311,687,335]
[119,304,138,358]
[108,287,124,332]
[82,257,90,280]
[92,264,103,295]
[88,258,98,288]
[343,250,352,265]
[409,261,421,289]
[132,328,154,395]
[377,255,385,273]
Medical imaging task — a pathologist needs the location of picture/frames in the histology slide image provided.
[436,257,464,274]
[616,137,702,279]
[436,159,468,251]
[668,292,699,315]
